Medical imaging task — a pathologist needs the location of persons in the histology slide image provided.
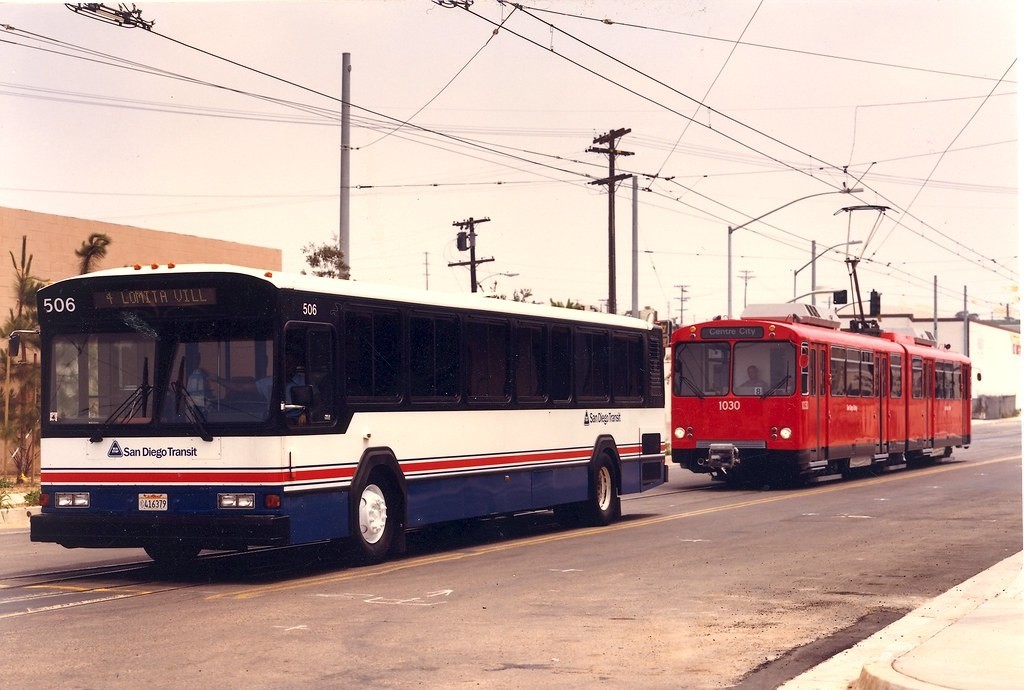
[740,365,769,389]
[206,349,309,426]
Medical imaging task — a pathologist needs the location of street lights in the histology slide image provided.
[727,187,866,321]
[793,240,865,305]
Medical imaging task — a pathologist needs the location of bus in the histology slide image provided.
[668,303,982,493]
[9,263,672,571]
